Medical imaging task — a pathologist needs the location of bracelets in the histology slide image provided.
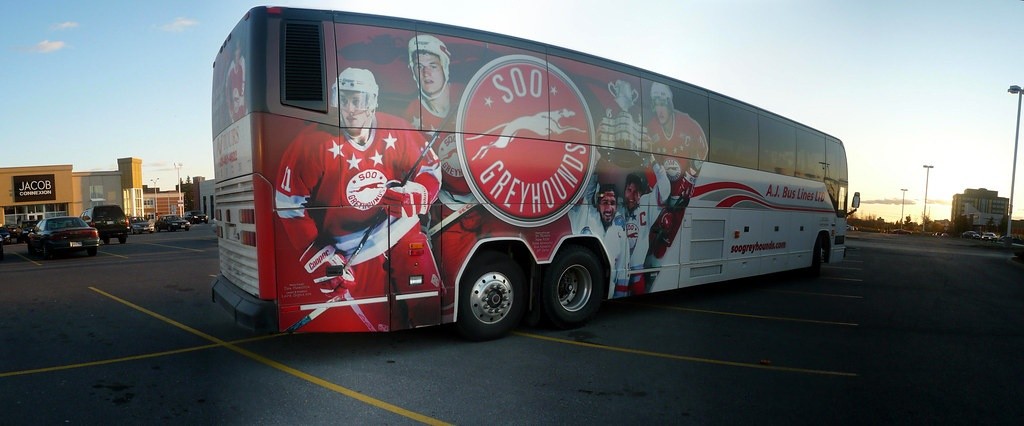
[651,159,658,167]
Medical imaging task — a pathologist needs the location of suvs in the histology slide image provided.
[79,204,131,246]
[184,210,209,224]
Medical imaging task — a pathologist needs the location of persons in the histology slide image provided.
[403,34,522,322]
[225,38,248,123]
[276,67,453,330]
[569,82,708,298]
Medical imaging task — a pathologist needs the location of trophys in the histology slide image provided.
[596,79,651,167]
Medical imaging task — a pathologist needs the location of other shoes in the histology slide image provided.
[654,245,666,258]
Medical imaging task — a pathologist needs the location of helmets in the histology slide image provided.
[332,67,378,109]
[650,82,673,105]
[408,35,451,81]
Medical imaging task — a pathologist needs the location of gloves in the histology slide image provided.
[382,180,429,219]
[299,238,357,300]
[668,171,697,211]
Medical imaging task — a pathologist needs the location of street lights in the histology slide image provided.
[921,165,934,233]
[150,177,159,221]
[1004,85,1024,249]
[900,188,908,229]
[173,162,185,218]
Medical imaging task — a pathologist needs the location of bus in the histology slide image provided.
[211,4,860,343]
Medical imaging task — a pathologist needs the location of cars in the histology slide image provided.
[941,233,950,238]
[27,215,101,261]
[128,217,155,235]
[1000,235,1006,242]
[155,214,192,233]
[962,231,981,240]
[934,231,942,238]
[891,228,913,237]
[0,218,39,263]
[847,224,859,232]
[981,232,999,243]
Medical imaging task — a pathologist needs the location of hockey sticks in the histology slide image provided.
[344,100,459,272]
[282,195,478,334]
